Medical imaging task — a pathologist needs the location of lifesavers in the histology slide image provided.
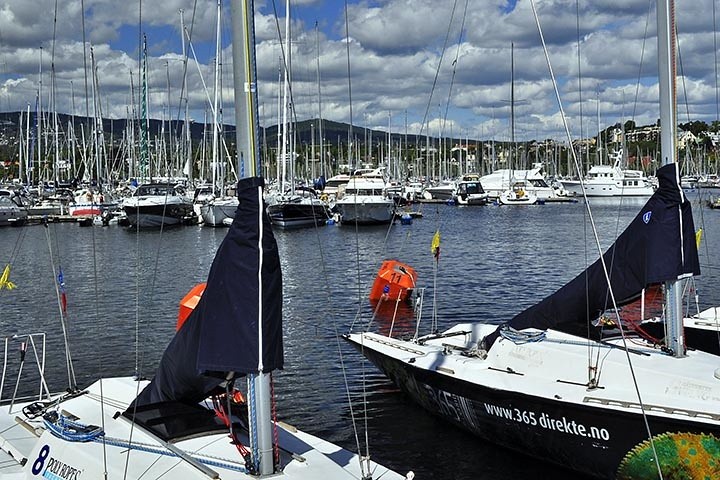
[321,195,328,204]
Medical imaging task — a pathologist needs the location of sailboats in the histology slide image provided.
[1,1,419,480]
[1,0,719,236]
[339,1,719,480]
